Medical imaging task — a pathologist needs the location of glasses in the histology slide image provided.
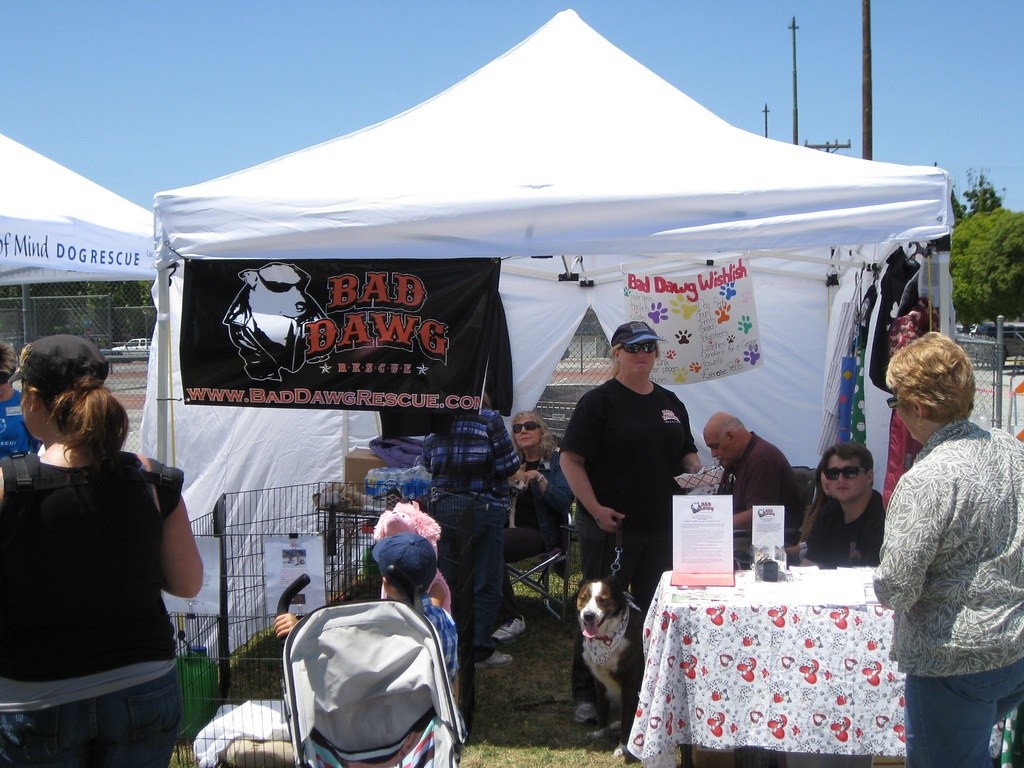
[0,371,15,384]
[513,421,540,434]
[887,397,905,408]
[824,466,866,480]
[621,342,656,353]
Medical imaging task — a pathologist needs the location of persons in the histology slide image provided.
[703,411,802,531]
[0,334,202,768]
[273,500,461,682]
[786,442,888,568]
[874,332,1024,768]
[559,320,702,724]
[493,410,573,641]
[421,390,520,670]
[0,345,42,458]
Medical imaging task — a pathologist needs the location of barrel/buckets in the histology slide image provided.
[175,645,218,739]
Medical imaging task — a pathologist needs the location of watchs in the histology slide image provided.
[537,475,545,483]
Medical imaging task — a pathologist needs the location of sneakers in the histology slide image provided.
[491,615,525,640]
[574,701,597,722]
[474,650,513,668]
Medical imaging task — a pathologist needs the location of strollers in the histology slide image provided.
[272,565,467,768]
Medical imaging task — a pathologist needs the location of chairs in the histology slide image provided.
[734,465,818,571]
[506,502,573,622]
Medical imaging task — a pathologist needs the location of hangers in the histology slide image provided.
[872,273,876,285]
[907,242,925,260]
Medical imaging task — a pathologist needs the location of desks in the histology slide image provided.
[627,568,1007,768]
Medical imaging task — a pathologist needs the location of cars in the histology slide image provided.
[110,338,152,355]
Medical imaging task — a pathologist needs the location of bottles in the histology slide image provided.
[364,466,427,514]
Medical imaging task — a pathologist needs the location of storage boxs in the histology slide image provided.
[345,447,386,495]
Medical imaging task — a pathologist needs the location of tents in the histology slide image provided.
[0,132,159,286]
[135,6,957,620]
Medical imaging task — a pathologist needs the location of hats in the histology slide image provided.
[372,532,436,591]
[7,334,109,394]
[611,321,662,347]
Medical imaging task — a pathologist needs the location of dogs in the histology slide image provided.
[576,571,647,756]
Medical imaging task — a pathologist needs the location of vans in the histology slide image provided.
[974,322,1024,368]
